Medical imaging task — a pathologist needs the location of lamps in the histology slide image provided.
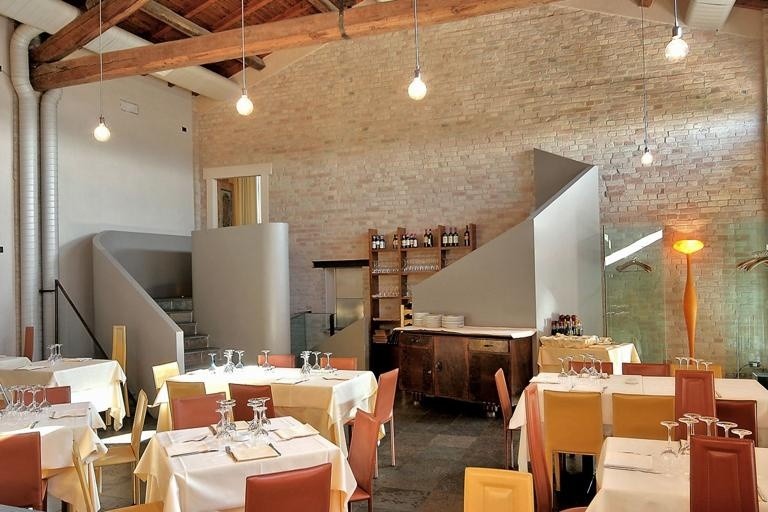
[663,0,692,61]
[638,0,655,166]
[671,238,705,367]
[234,0,256,117]
[406,0,429,102]
[91,1,112,142]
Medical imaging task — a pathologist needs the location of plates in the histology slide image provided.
[165,441,217,455]
[529,375,559,383]
[601,449,653,471]
[441,314,465,328]
[271,373,355,386]
[230,443,277,461]
[276,423,320,439]
[424,314,441,327]
[48,409,88,420]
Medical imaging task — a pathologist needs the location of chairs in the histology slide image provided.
[464,338,768,512]
[0,321,398,512]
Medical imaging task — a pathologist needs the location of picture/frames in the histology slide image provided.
[217,178,236,228]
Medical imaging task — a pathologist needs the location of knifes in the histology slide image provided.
[170,449,219,457]
[268,442,281,455]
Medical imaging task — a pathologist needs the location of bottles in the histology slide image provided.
[551,314,583,336]
[372,224,470,249]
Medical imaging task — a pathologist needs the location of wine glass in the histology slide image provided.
[214,395,271,446]
[0,343,64,430]
[557,353,607,390]
[377,286,410,297]
[675,356,713,371]
[656,413,753,476]
[371,256,440,273]
[208,350,334,379]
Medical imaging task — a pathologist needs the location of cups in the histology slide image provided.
[413,313,424,326]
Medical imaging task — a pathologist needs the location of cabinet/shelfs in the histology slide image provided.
[368,224,479,378]
[393,325,536,417]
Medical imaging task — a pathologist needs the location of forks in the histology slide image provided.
[183,435,208,442]
[225,445,237,461]
